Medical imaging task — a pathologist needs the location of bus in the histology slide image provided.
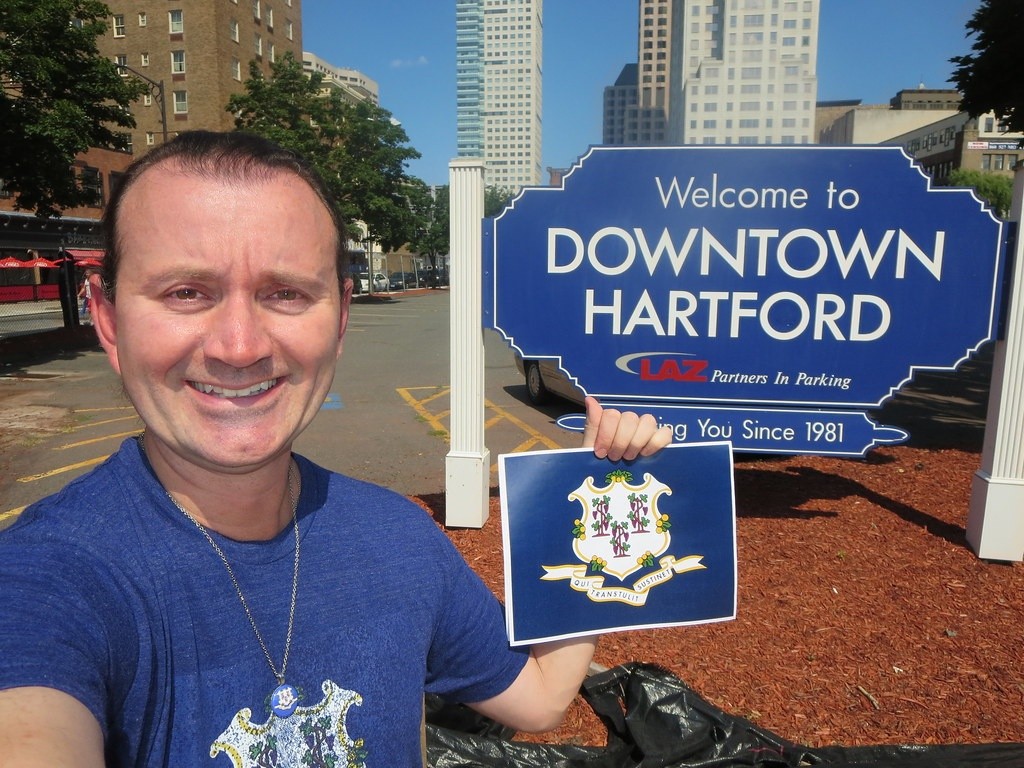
[349,250,365,277]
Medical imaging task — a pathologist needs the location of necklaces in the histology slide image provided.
[135,430,305,718]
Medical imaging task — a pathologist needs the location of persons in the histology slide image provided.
[0,127,675,768]
[75,268,95,318]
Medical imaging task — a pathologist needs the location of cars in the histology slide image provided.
[359,272,389,291]
[416,267,449,287]
[512,349,585,408]
[389,272,416,290]
